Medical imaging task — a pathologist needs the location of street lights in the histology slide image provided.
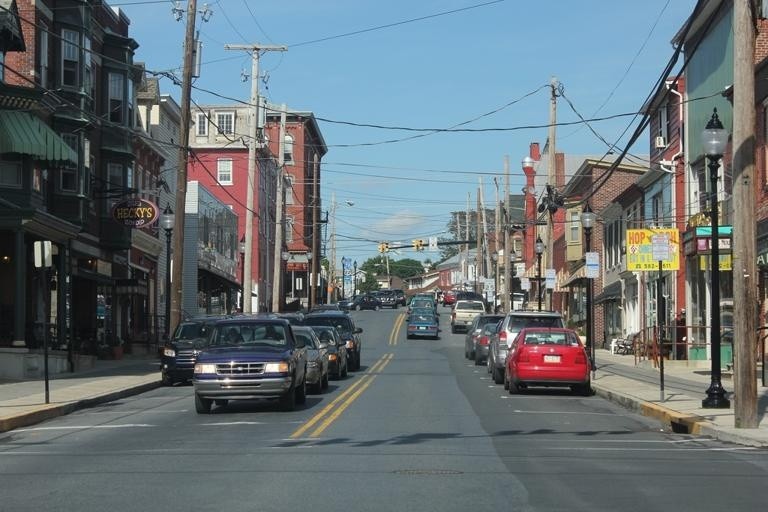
[508,246,515,310]
[279,244,290,309]
[341,256,345,300]
[703,103,732,414]
[491,249,498,312]
[353,260,357,296]
[472,257,481,295]
[533,233,545,311]
[158,200,176,352]
[374,253,391,289]
[327,190,354,306]
[580,202,599,371]
[236,231,247,311]
[306,246,312,312]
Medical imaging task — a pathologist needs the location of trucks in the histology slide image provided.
[451,301,486,334]
[407,292,438,318]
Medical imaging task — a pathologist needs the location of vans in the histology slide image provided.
[66,276,70,330]
[438,289,483,306]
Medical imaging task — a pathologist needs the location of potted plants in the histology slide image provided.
[112,336,125,360]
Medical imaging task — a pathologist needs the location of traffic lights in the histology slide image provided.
[378,245,381,251]
[419,240,423,249]
[412,240,415,250]
[385,242,389,252]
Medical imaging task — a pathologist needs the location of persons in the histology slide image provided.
[224,328,245,345]
[262,325,284,342]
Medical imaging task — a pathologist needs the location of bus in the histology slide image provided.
[718,297,733,342]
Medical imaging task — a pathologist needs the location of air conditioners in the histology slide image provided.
[654,136,664,148]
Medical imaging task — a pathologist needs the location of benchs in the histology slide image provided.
[616,333,637,355]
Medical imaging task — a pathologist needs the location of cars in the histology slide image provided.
[405,308,439,339]
[97,294,105,324]
[501,327,591,396]
[163,302,364,414]
[337,294,382,313]
[464,309,564,384]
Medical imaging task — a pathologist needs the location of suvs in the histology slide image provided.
[378,289,397,309]
[492,293,525,310]
[391,289,407,307]
